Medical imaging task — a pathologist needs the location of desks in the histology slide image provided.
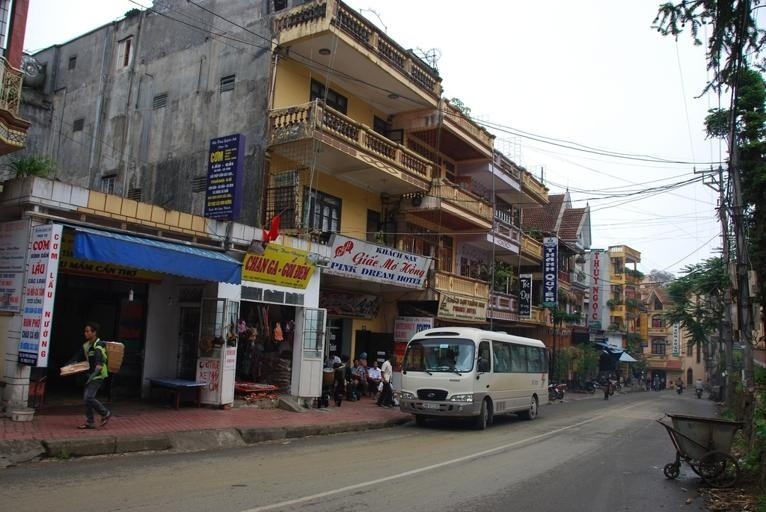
[147,376,207,410]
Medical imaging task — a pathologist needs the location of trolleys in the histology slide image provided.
[656,412,746,489]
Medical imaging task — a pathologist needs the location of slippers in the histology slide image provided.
[99,410,111,426]
[77,422,96,429]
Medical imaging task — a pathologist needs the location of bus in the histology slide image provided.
[400,326,549,430]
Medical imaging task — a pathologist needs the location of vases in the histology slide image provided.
[10,410,36,422]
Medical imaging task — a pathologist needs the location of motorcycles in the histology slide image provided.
[578,375,624,394]
[548,384,568,401]
[695,388,702,399]
[676,384,683,395]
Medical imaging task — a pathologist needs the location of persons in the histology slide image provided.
[327,351,398,407]
[76,321,113,430]
[653,374,704,395]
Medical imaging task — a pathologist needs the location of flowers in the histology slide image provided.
[6,400,27,409]
